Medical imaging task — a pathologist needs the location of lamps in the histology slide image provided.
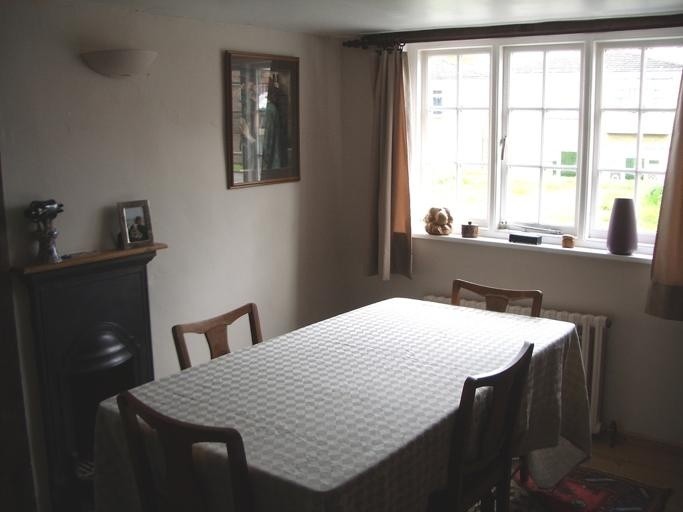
[79,45,159,85]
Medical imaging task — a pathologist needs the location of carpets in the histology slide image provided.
[460,454,680,512]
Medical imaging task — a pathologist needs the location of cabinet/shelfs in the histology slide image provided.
[0,237,170,512]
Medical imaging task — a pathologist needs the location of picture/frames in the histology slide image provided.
[224,48,301,190]
[115,199,155,247]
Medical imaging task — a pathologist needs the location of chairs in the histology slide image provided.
[172,301,263,372]
[113,388,254,511]
[449,278,544,317]
[427,340,534,512]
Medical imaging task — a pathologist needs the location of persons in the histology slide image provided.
[239,78,282,170]
[129,216,146,241]
